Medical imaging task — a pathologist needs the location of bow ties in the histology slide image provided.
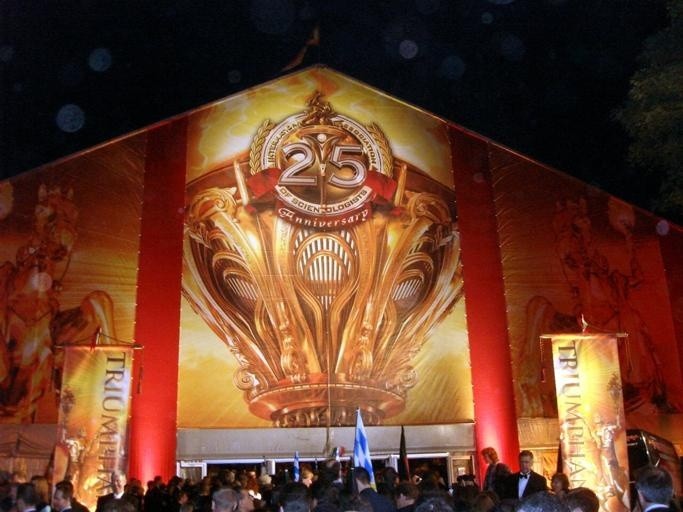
[520,474,527,479]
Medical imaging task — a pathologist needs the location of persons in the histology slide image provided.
[630,466,681,512]
[0,447,599,511]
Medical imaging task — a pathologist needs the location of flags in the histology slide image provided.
[355,411,378,493]
[399,430,411,483]
